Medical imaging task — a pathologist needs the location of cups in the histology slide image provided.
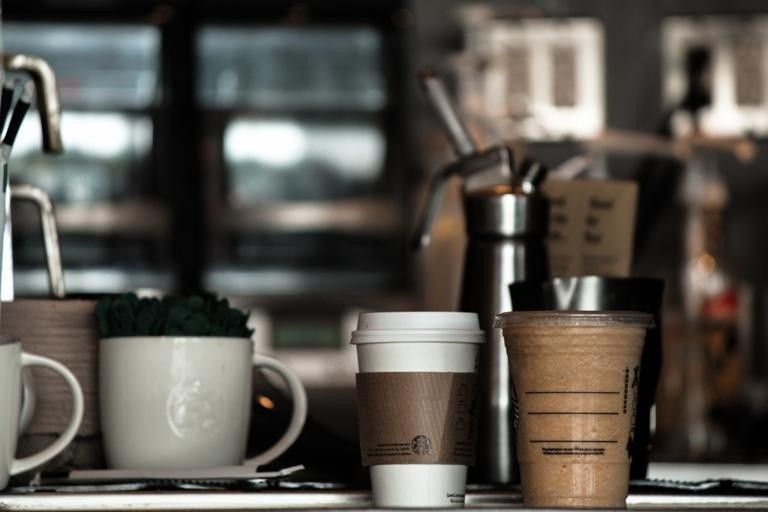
[493,310,656,510]
[97,335,307,471]
[348,311,486,512]
[506,275,666,482]
[0,334,86,493]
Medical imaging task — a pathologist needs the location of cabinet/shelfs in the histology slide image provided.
[0,3,421,314]
